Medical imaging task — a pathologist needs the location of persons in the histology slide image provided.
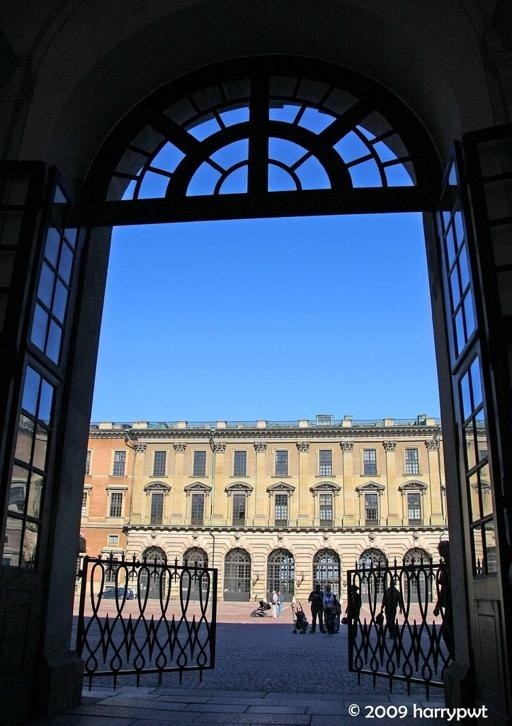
[344,585,363,638]
[307,582,341,636]
[277,590,284,618]
[270,588,278,618]
[433,541,453,661]
[380,579,404,639]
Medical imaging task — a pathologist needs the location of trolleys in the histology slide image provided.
[290,602,313,635]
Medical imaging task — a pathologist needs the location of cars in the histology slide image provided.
[98,588,134,600]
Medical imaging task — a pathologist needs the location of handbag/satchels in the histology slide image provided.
[341,617,348,624]
[375,612,384,625]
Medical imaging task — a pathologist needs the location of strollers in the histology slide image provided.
[250,601,271,617]
